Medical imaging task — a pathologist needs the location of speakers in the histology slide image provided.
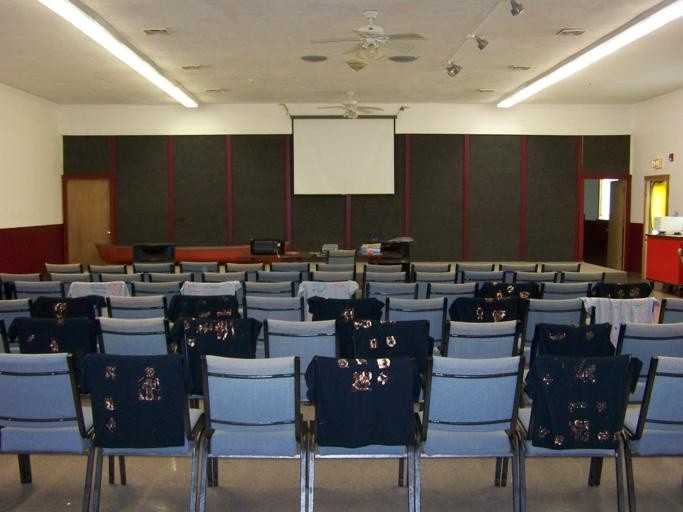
[133,242,176,263]
[382,241,416,259]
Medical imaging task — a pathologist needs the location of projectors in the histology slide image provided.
[250,238,286,255]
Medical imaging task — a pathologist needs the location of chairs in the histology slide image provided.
[0,237,681,512]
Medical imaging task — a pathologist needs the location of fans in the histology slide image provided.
[307,11,422,54]
[315,93,389,118]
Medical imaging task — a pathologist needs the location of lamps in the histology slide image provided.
[360,41,383,62]
[40,0,198,113]
[446,1,525,80]
[343,110,357,121]
[493,1,682,113]
